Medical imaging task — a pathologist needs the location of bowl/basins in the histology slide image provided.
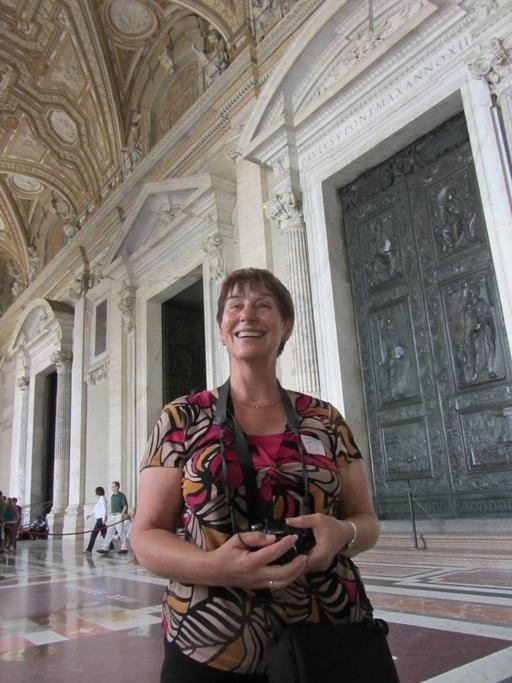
[232,396,282,408]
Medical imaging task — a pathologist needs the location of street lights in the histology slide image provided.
[265,618,400,683]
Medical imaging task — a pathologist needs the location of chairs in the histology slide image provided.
[97,549,128,555]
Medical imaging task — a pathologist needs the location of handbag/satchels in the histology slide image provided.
[339,519,357,555]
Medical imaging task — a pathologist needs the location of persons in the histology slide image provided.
[98,481,128,555]
[131,268,379,682]
[82,486,115,554]
[1,491,48,553]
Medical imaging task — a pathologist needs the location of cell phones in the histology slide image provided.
[250,524,316,566]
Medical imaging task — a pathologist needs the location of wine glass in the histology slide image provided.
[269,580,274,589]
